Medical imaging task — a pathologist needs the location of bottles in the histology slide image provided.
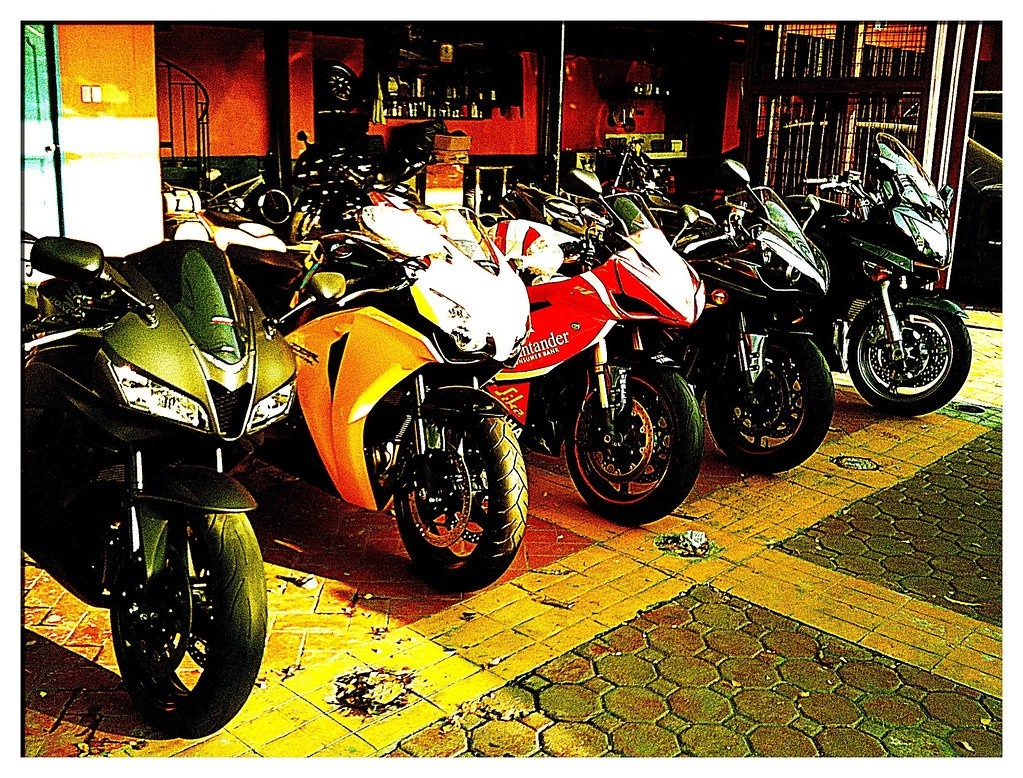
[384,85,497,118]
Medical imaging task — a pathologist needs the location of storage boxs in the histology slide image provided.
[423,134,473,211]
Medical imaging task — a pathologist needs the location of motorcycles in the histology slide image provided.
[20,131,971,740]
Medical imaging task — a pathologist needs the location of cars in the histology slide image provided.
[722,121,1002,304]
